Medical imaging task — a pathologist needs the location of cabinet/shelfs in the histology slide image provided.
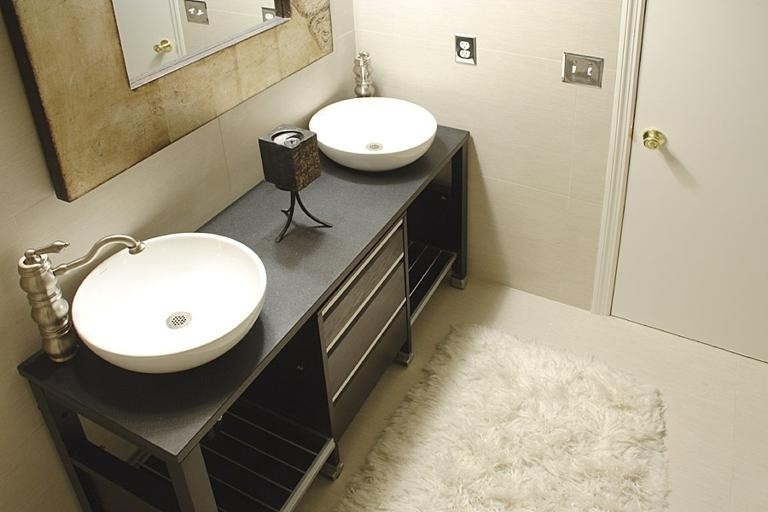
[17,131,468,512]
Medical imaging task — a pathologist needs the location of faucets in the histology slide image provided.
[15,233,147,364]
[352,49,376,98]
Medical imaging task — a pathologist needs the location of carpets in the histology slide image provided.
[331,321,669,510]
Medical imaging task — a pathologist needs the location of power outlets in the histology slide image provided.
[455,36,476,65]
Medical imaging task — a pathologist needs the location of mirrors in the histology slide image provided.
[109,0,293,90]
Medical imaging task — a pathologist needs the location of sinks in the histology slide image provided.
[306,96,439,174]
[70,231,269,376]
